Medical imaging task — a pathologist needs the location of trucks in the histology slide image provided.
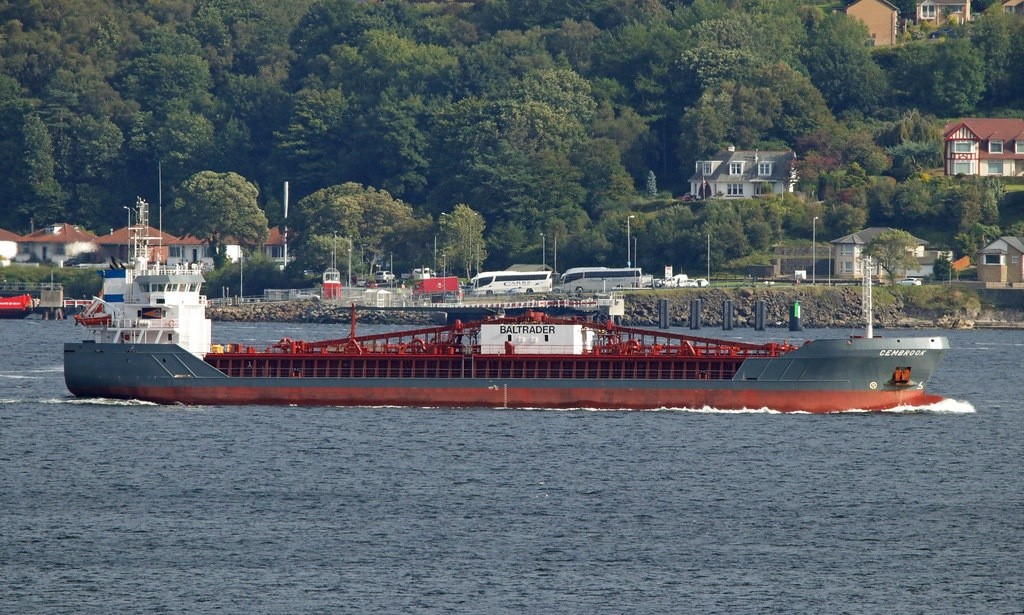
[413,276,460,304]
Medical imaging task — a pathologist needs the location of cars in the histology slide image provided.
[296,292,321,302]
[643,273,710,288]
[896,277,923,287]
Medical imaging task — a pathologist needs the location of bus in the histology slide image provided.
[471,270,553,297]
[559,266,642,293]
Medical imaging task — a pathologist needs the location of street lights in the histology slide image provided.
[123,206,131,264]
[539,232,546,271]
[361,244,367,275]
[347,234,354,287]
[627,214,635,268]
[440,212,451,275]
[333,230,339,272]
[157,159,165,262]
[330,251,334,301]
[812,216,819,285]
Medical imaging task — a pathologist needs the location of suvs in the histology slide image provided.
[375,270,396,283]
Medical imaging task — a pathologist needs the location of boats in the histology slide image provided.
[58,193,951,416]
[1,292,41,320]
[73,291,112,327]
